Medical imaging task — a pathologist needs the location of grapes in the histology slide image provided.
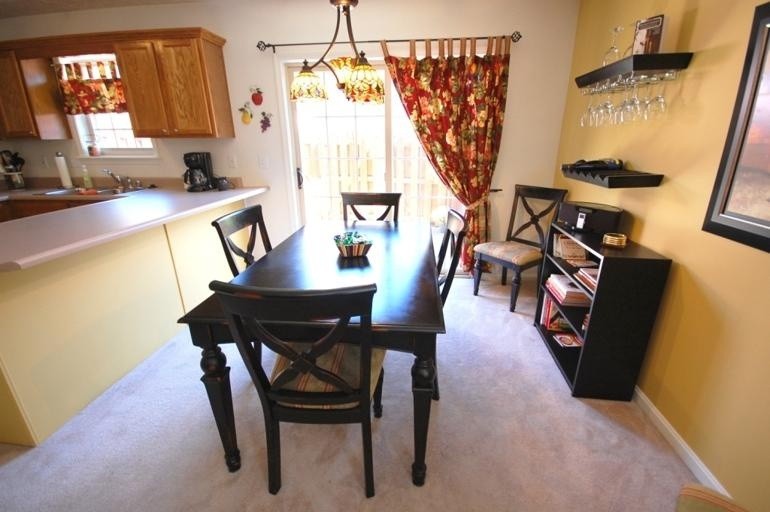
[260,115,271,134]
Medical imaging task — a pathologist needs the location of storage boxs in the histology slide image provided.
[559,202,624,236]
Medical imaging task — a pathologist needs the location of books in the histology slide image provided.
[540,233,599,352]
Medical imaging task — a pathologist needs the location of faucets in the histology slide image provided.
[102,168,123,187]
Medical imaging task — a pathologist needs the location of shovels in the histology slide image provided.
[1,149,25,173]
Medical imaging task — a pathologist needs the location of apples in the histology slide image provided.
[252,90,263,106]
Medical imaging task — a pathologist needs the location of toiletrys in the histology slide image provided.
[82,166,94,189]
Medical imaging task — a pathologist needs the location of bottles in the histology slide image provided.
[86,140,101,156]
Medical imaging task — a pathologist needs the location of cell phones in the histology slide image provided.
[576,212,586,229]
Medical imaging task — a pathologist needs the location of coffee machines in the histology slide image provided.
[182,152,216,192]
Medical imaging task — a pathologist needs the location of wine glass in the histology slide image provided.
[601,25,625,66]
[577,70,676,130]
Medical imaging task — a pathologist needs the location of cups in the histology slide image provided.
[217,178,228,191]
[3,171,25,190]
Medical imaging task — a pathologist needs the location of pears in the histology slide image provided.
[239,108,252,124]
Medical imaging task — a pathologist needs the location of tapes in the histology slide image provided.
[603,233,627,248]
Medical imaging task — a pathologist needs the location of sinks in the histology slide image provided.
[46,189,83,195]
[97,188,138,195]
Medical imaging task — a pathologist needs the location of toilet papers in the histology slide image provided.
[55,156,73,189]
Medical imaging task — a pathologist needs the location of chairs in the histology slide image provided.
[212,204,273,277]
[436,210,469,305]
[472,183,568,312]
[209,280,385,499]
[341,191,401,221]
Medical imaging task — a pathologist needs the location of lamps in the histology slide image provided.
[289,0,384,103]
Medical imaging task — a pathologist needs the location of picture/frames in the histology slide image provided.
[702,3,770,253]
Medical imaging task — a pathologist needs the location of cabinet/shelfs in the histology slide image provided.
[561,53,693,189]
[534,221,672,401]
[111,38,235,139]
[0,50,73,141]
